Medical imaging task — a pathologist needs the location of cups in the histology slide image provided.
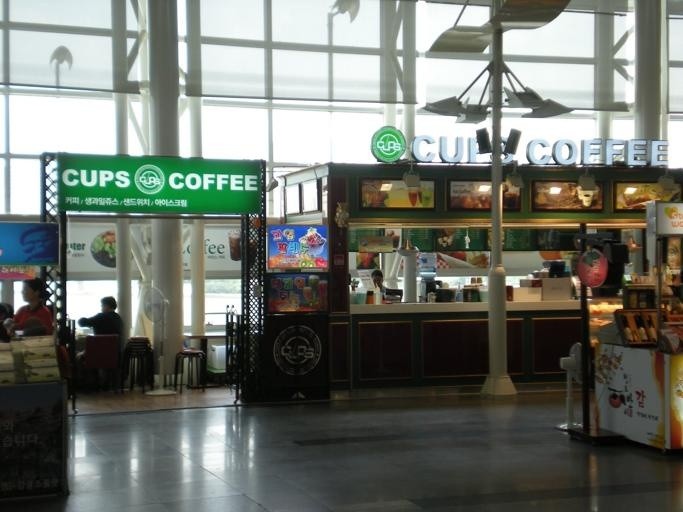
[228,229,241,260]
[581,192,593,206]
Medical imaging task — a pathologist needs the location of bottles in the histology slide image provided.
[367,291,374,305]
[621,313,657,342]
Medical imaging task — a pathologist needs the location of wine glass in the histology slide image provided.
[303,275,328,305]
[407,185,433,207]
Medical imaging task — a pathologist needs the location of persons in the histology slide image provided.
[78,296,122,337]
[372,269,386,292]
[0,275,55,342]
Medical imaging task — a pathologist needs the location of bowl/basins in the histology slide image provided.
[298,237,327,255]
[532,271,548,278]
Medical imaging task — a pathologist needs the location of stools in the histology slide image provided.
[172,348,207,396]
[123,336,155,396]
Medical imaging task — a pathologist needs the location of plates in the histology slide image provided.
[91,232,116,268]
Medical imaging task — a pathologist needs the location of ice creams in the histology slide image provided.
[299,227,325,256]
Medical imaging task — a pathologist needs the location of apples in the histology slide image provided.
[608,393,621,409]
[575,248,609,288]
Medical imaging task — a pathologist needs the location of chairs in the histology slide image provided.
[80,334,123,395]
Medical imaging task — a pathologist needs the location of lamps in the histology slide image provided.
[411,1,572,124]
